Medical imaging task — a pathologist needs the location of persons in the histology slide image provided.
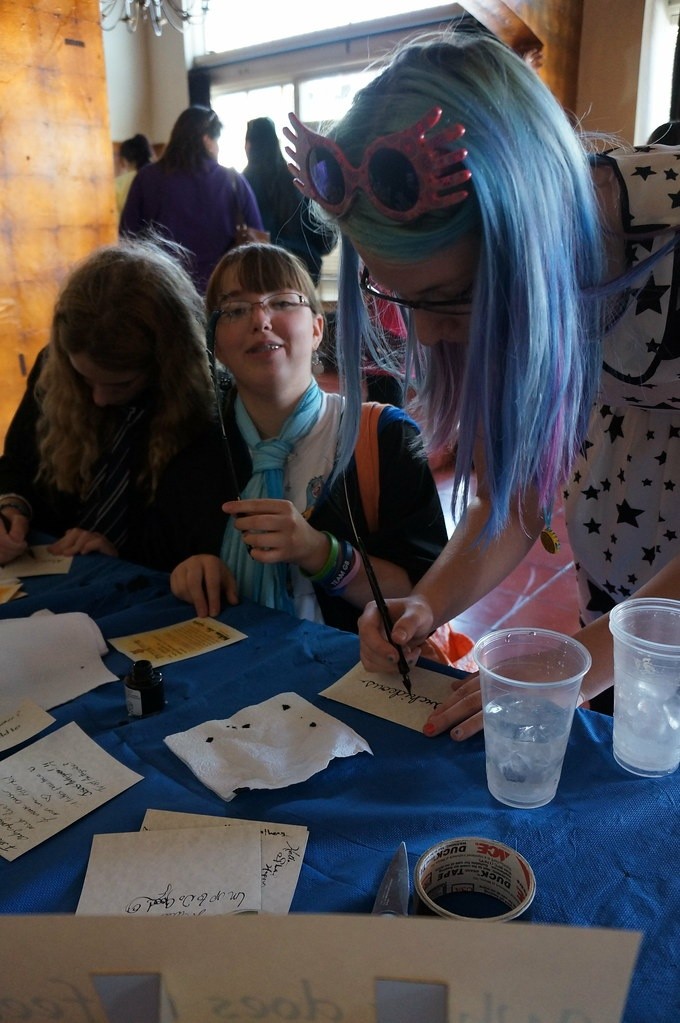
[0,244,230,566]
[168,241,450,630]
[308,25,680,742]
[241,118,321,287]
[119,107,262,298]
[115,132,156,214]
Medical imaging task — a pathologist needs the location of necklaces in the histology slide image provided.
[542,497,560,555]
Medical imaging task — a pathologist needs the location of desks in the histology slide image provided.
[0,530,680,1023]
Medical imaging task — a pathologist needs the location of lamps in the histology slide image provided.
[96,0,210,36]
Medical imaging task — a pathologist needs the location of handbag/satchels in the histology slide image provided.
[306,202,338,254]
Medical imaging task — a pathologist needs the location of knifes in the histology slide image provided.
[371,841,410,919]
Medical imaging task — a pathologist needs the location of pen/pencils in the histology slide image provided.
[0,511,36,560]
[204,308,255,561]
[339,466,414,701]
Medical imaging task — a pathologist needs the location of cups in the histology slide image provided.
[608,598,680,777]
[413,837,536,925]
[472,627,593,810]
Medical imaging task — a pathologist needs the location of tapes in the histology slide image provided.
[411,835,537,925]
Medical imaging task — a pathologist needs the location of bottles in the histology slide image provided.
[124,660,164,719]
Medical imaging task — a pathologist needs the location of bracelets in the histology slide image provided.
[299,531,361,597]
[0,502,20,510]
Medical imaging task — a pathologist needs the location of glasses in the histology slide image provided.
[283,105,471,221]
[212,293,314,321]
[359,264,473,315]
